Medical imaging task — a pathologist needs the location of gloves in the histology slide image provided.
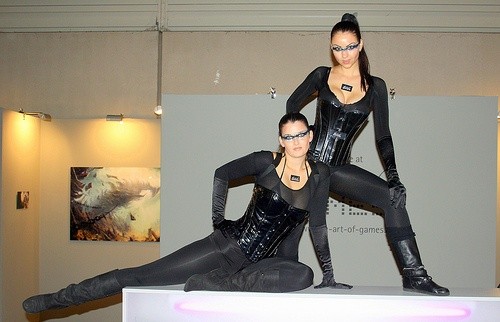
[307,148,321,163]
[211,177,242,239]
[375,135,406,208]
[308,225,335,288]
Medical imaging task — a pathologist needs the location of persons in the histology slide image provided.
[22,112,353,314]
[285,13,451,297]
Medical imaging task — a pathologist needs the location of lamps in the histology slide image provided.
[18,107,52,121]
[106,115,122,121]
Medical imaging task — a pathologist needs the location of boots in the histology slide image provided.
[389,236,451,295]
[184,257,279,292]
[22,269,124,312]
